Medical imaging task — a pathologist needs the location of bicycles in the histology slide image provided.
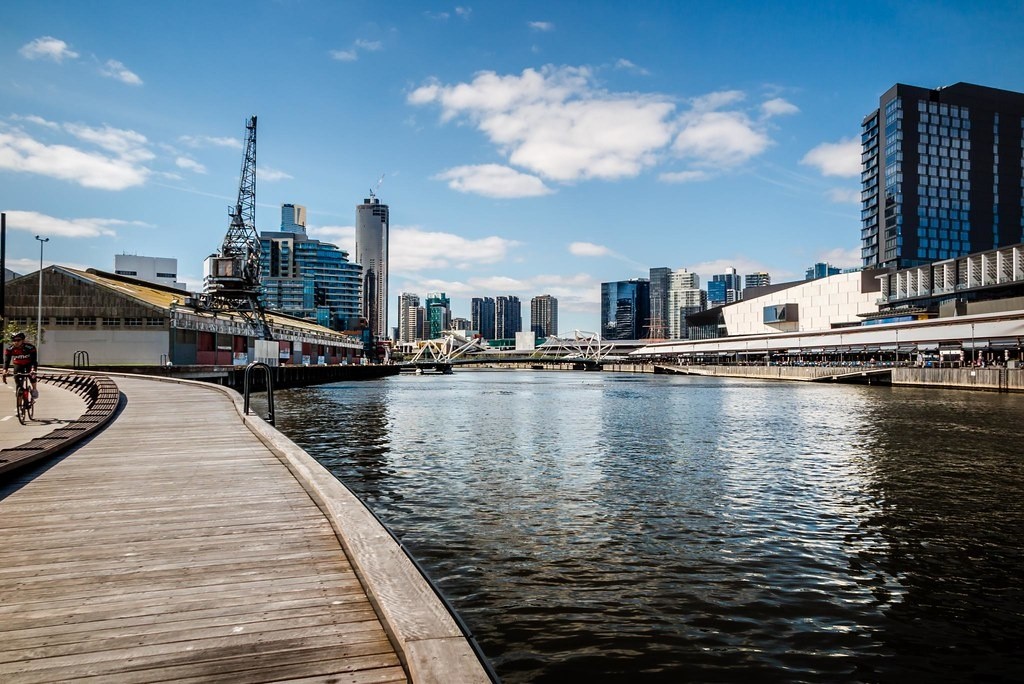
[2,372,35,425]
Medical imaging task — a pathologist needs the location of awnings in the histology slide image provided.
[897,346,916,355]
[801,347,880,354]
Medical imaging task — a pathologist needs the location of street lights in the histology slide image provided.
[34,235,48,368]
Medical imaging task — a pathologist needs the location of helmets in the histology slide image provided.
[11,331,25,340]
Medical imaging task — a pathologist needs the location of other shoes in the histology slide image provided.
[32,390,38,398]
[16,411,22,416]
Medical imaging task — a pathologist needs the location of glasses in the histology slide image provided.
[12,339,22,342]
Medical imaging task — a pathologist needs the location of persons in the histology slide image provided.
[917,351,923,368]
[940,359,945,368]
[870,357,876,369]
[979,358,986,368]
[905,359,908,367]
[927,360,932,368]
[1,331,38,417]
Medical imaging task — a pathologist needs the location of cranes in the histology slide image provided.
[208,113,266,287]
